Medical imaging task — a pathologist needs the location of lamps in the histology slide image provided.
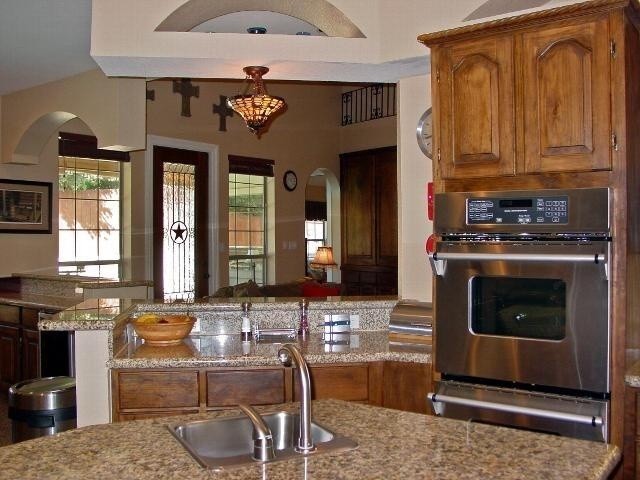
[225,66,287,136]
[311,247,336,283]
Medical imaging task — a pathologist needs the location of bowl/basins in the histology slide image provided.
[127,313,196,347]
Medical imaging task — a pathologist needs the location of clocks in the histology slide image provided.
[283,171,297,191]
[416,107,433,160]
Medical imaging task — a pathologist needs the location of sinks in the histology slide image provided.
[165,408,361,475]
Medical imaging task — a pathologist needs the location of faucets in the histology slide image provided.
[278,344,319,455]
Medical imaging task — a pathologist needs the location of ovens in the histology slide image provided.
[426,188,613,443]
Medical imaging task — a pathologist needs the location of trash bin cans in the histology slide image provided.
[8,376,77,443]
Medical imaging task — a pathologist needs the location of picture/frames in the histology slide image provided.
[0,178,53,235]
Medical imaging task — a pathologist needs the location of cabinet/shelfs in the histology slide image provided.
[111,361,437,422]
[337,145,398,296]
[416,0,640,180]
[0,303,39,393]
